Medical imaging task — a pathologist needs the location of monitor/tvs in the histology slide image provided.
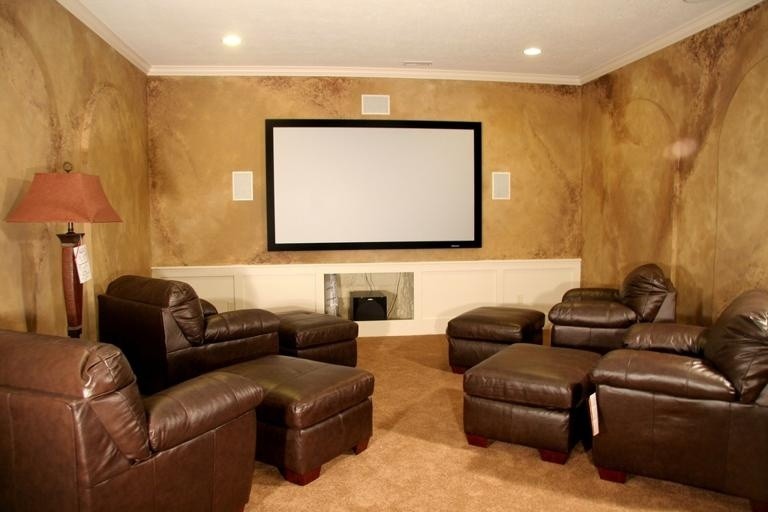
[263,117,482,251]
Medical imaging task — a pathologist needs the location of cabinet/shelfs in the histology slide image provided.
[149,258,581,338]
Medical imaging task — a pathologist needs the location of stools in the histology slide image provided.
[222,309,376,485]
[446,305,602,466]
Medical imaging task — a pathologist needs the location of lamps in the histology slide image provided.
[5,170,124,337]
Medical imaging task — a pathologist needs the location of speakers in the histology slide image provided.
[353,296,387,320]
